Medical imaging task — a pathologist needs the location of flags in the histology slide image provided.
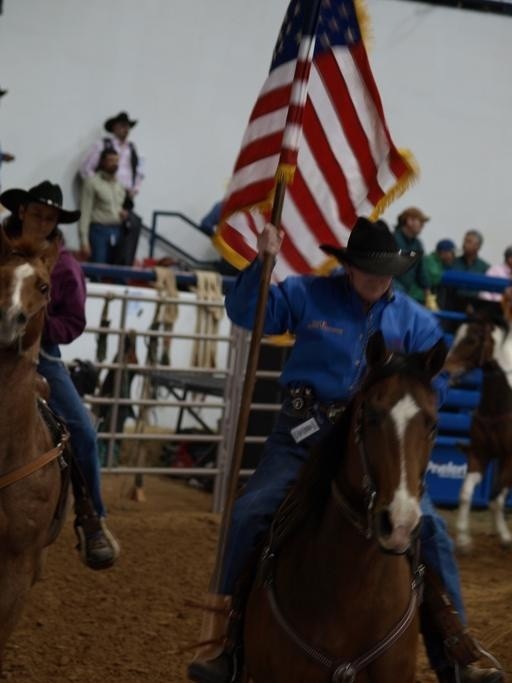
[211,0,416,339]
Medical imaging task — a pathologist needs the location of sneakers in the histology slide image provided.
[71,514,121,569]
[437,662,502,683]
[185,641,242,680]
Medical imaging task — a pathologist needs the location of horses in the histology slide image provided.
[245,329,452,683]
[443,303,512,556]
[0,227,62,673]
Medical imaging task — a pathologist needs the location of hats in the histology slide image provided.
[318,215,424,277]
[104,111,137,130]
[0,180,81,222]
[401,206,431,222]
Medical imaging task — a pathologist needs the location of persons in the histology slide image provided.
[77,112,145,287]
[1,180,117,571]
[392,206,512,335]
